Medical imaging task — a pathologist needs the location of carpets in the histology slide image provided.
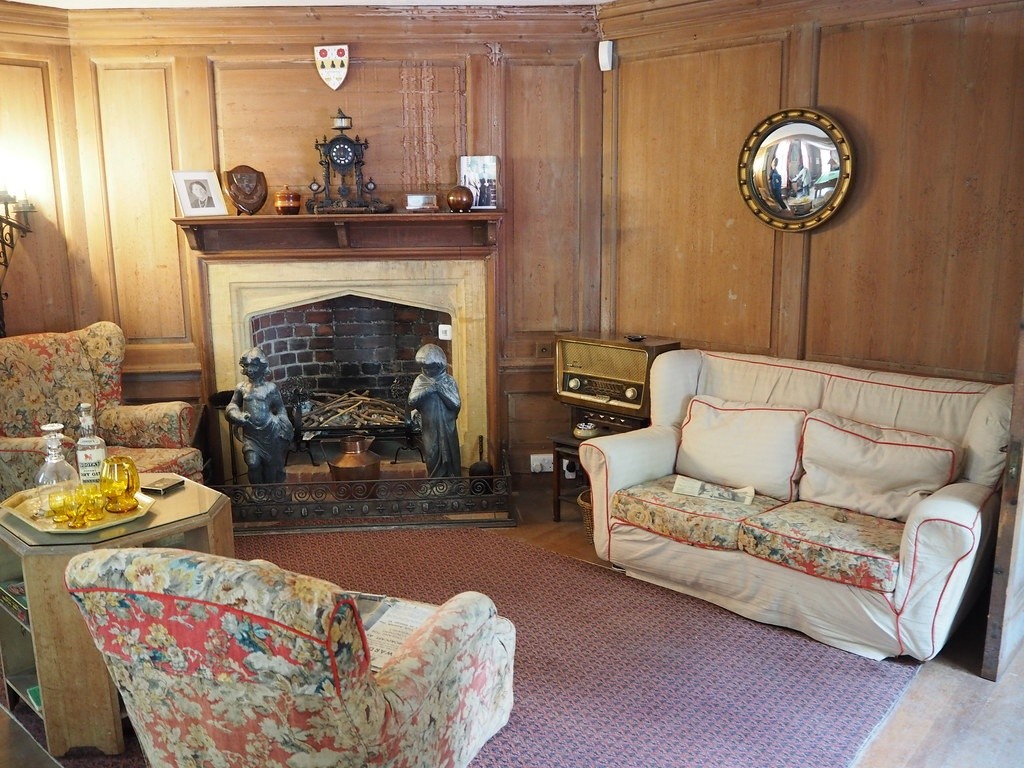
[0,525,923,768]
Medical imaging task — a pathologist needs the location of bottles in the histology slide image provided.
[274,185,301,214]
[75,403,107,485]
[574,415,600,440]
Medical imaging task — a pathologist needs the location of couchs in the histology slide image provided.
[578,348,1015,661]
[63,547,516,768]
[0,321,205,547]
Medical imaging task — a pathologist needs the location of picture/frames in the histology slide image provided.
[170,169,229,218]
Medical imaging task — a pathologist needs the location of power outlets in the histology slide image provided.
[530,454,577,480]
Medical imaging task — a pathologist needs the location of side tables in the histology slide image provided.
[0,472,234,758]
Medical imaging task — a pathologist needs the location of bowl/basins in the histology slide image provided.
[624,335,647,341]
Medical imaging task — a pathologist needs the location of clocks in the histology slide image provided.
[307,108,394,215]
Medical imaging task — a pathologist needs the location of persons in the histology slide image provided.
[189,181,215,208]
[407,344,461,482]
[768,157,811,209]
[225,347,293,496]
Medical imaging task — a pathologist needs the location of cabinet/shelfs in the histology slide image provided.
[546,401,649,523]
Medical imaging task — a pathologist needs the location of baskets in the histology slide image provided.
[577,490,594,545]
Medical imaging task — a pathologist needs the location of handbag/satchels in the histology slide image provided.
[801,182,803,187]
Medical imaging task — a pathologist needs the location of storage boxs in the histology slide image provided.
[0,578,29,625]
[141,477,186,495]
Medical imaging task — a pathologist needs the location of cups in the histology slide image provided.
[406,193,438,213]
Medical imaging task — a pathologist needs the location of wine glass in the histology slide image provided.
[34,422,79,517]
[48,484,106,527]
[99,456,141,513]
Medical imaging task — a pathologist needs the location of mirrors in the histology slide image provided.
[736,107,854,232]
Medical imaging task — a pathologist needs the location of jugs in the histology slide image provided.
[319,434,380,498]
